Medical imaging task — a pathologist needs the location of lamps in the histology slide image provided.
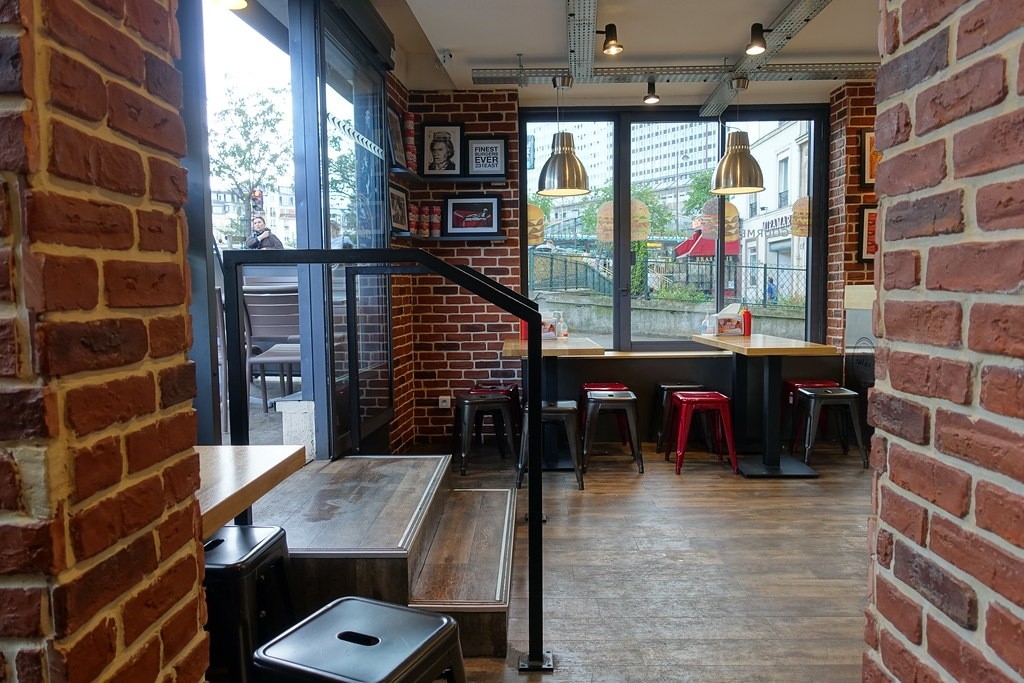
[643,82,659,103]
[709,77,765,195]
[595,23,623,55]
[746,23,773,56]
[536,76,590,197]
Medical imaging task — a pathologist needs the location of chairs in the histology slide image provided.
[211,275,298,434]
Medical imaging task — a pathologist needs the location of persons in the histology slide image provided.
[329,223,354,277]
[245,216,285,250]
[767,277,778,306]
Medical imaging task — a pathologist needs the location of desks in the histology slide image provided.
[500,338,604,470]
[189,444,307,539]
[693,333,837,478]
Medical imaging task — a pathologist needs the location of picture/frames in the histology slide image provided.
[385,101,408,169]
[442,194,501,237]
[860,128,875,189]
[418,122,466,177]
[387,182,410,232]
[857,204,878,264]
[465,136,509,178]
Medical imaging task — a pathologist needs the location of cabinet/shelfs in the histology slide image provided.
[389,167,507,241]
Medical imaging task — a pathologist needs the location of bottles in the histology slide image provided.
[743,306,751,336]
[521,319,528,340]
[557,310,567,338]
[739,306,746,315]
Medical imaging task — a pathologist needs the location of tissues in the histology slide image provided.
[541,311,558,340]
[710,302,744,336]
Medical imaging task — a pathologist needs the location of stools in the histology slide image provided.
[253,596,467,683]
[201,525,294,683]
[652,382,739,474]
[781,378,869,469]
[452,381,644,491]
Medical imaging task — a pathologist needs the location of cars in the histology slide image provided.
[529,244,583,253]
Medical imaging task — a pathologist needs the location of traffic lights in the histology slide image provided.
[252,190,263,211]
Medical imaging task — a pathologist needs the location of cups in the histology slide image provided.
[404,112,418,174]
[408,204,442,237]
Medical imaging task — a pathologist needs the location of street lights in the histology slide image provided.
[572,209,579,250]
[676,152,690,237]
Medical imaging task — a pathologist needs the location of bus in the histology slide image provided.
[542,235,688,257]
